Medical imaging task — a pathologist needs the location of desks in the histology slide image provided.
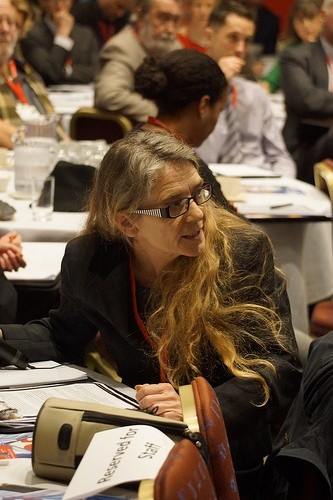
[0,85,333,306]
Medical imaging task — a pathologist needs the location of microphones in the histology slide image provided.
[0,339,30,369]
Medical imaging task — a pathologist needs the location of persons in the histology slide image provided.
[0,0,333,364]
[0,129,305,500]
[255,330,333,500]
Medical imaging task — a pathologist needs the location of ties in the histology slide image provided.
[217,103,242,164]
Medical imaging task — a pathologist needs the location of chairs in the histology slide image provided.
[314,159,333,202]
[70,108,131,145]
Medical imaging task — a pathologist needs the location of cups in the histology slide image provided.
[14,144,50,200]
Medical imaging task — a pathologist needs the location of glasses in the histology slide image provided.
[116,182,213,219]
